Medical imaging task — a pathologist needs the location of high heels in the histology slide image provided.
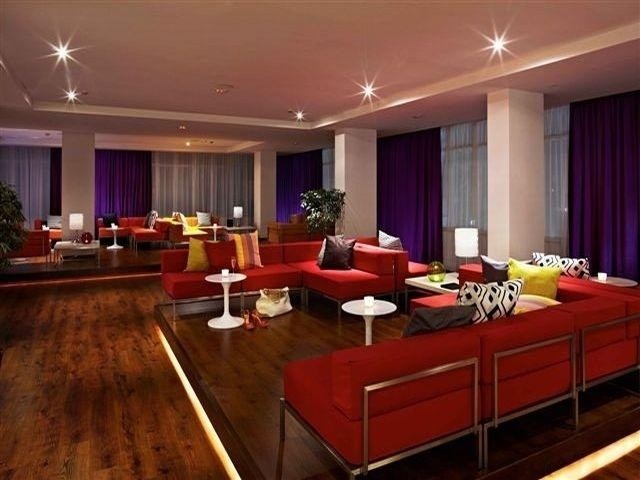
[252,309,269,329]
[241,308,255,332]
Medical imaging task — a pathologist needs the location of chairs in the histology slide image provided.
[10,217,169,263]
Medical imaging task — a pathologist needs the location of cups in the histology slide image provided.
[222,269,229,277]
[213,223,218,228]
[597,272,607,280]
[365,296,374,308]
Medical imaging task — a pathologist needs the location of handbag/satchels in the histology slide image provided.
[254,286,294,319]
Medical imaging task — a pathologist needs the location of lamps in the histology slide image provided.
[454,227,480,265]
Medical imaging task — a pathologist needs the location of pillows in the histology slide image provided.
[480,255,533,284]
[402,303,478,337]
[226,231,264,269]
[454,276,524,324]
[508,256,562,301]
[532,251,594,281]
[195,210,211,226]
[171,211,187,228]
[317,236,343,261]
[377,230,403,251]
[203,238,239,269]
[321,234,356,269]
[186,234,214,273]
[144,210,158,229]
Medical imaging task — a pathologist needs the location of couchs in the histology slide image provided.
[352,233,440,314]
[160,235,397,328]
[277,263,640,479]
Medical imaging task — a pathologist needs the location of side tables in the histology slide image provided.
[205,274,248,328]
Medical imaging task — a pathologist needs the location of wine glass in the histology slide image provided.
[231,257,236,277]
